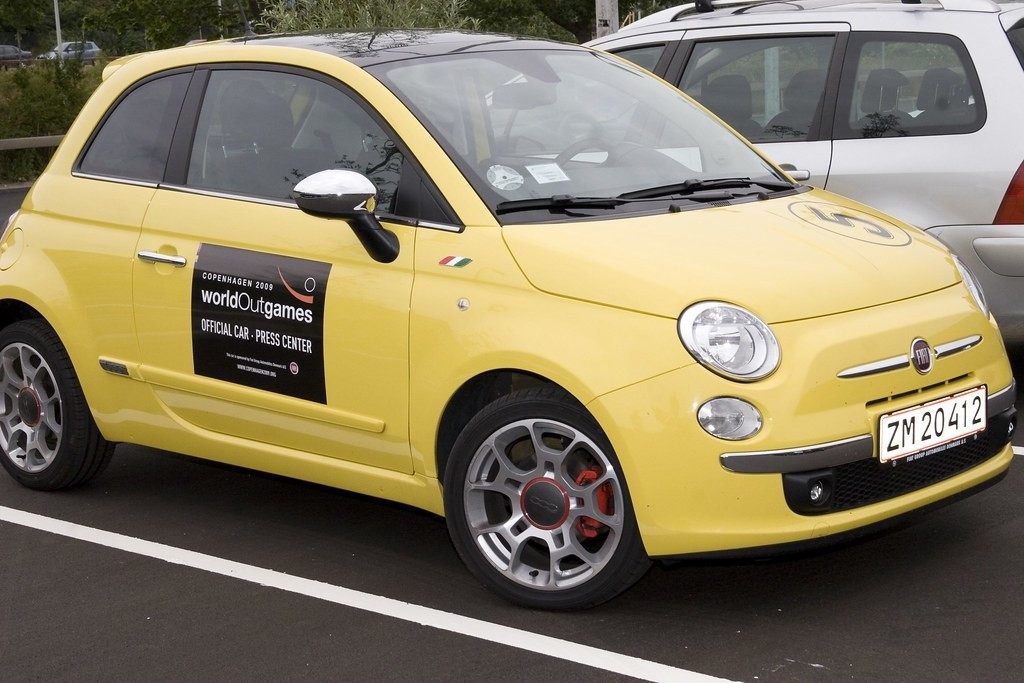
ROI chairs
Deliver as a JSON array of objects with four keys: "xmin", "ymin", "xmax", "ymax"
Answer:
[
  {"xmin": 700, "ymin": 69, "xmax": 827, "ymax": 141},
  {"xmin": 854, "ymin": 67, "xmax": 968, "ymax": 130},
  {"xmin": 215, "ymin": 94, "xmax": 322, "ymax": 198},
  {"xmin": 347, "ymin": 105, "xmax": 449, "ymax": 190}
]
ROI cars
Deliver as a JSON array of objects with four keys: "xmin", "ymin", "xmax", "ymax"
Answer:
[
  {"xmin": 0, "ymin": 29, "xmax": 1015, "ymax": 612},
  {"xmin": 36, "ymin": 41, "xmax": 103, "ymax": 60},
  {"xmin": 0, "ymin": 45, "xmax": 33, "ymax": 69}
]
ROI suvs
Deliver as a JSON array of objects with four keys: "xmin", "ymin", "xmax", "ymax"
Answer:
[{"xmin": 456, "ymin": 0, "xmax": 1024, "ymax": 367}]
